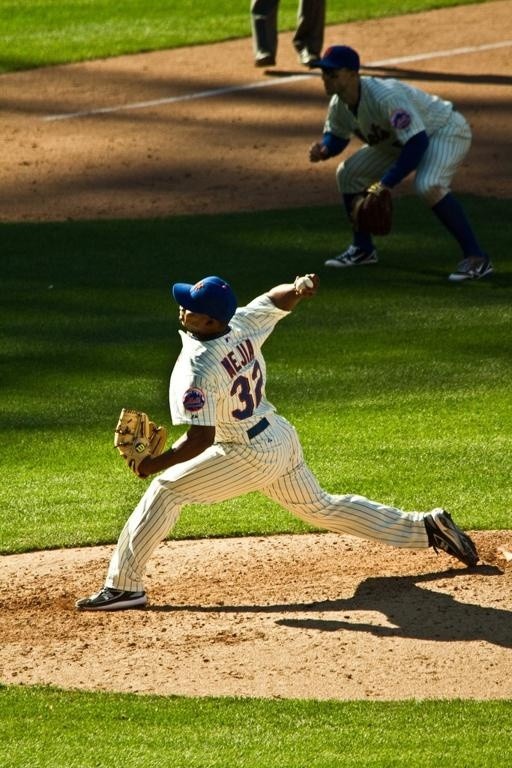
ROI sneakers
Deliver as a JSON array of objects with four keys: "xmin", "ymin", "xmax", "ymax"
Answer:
[
  {"xmin": 449, "ymin": 254, "xmax": 493, "ymax": 281},
  {"xmin": 423, "ymin": 507, "xmax": 479, "ymax": 567},
  {"xmin": 296, "ymin": 49, "xmax": 321, "ymax": 65},
  {"xmin": 254, "ymin": 54, "xmax": 276, "ymax": 68},
  {"xmin": 325, "ymin": 245, "xmax": 379, "ymax": 267},
  {"xmin": 74, "ymin": 586, "xmax": 148, "ymax": 612}
]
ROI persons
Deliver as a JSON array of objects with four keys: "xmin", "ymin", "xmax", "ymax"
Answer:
[
  {"xmin": 309, "ymin": 45, "xmax": 494, "ymax": 283},
  {"xmin": 75, "ymin": 272, "xmax": 479, "ymax": 611},
  {"xmin": 249, "ymin": 1, "xmax": 328, "ymax": 67}
]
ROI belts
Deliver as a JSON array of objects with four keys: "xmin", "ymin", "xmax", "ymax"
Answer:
[{"xmin": 248, "ymin": 416, "xmax": 270, "ymax": 440}]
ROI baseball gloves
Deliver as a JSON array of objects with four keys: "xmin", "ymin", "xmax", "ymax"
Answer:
[
  {"xmin": 352, "ymin": 179, "xmax": 391, "ymax": 235},
  {"xmin": 114, "ymin": 406, "xmax": 166, "ymax": 477}
]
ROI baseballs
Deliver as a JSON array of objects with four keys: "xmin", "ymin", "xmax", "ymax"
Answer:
[{"xmin": 295, "ymin": 276, "xmax": 314, "ymax": 292}]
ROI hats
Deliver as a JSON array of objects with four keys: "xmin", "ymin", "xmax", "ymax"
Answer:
[
  {"xmin": 172, "ymin": 275, "xmax": 238, "ymax": 324},
  {"xmin": 312, "ymin": 45, "xmax": 360, "ymax": 69}
]
